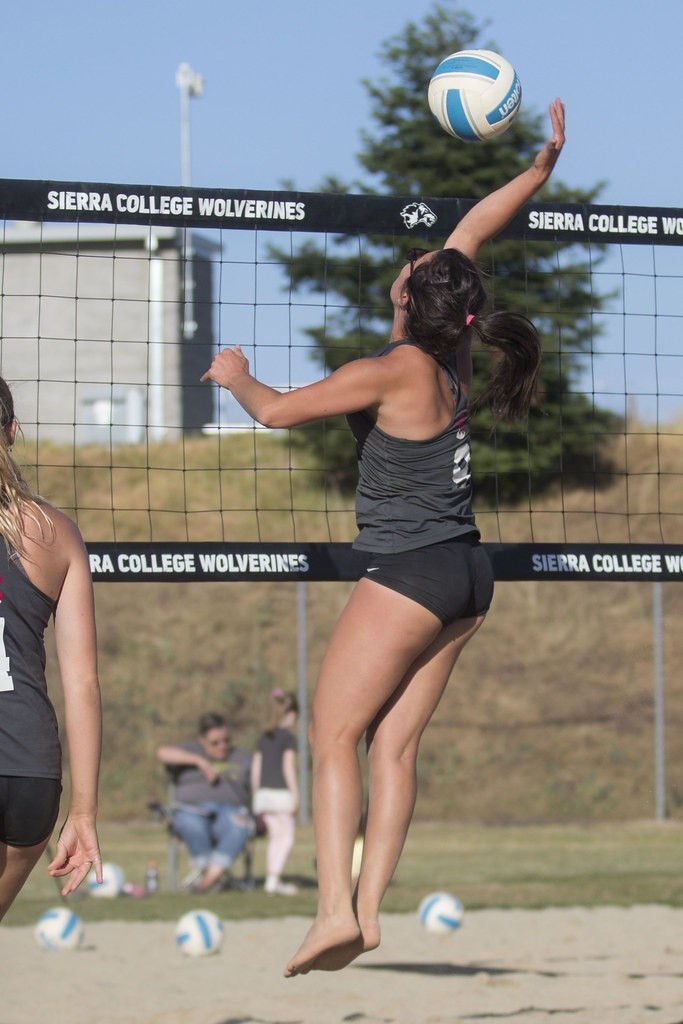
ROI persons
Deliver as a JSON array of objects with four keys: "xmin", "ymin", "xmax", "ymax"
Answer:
[
  {"xmin": 200, "ymin": 96, "xmax": 568, "ymax": 978},
  {"xmin": 1, "ymin": 377, "xmax": 107, "ymax": 924},
  {"xmin": 248, "ymin": 689, "xmax": 305, "ymax": 896},
  {"xmin": 156, "ymin": 709, "xmax": 256, "ymax": 893}
]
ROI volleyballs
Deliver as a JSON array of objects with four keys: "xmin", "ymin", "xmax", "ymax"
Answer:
[
  {"xmin": 35, "ymin": 908, "xmax": 84, "ymax": 951},
  {"xmin": 87, "ymin": 863, "xmax": 127, "ymax": 901},
  {"xmin": 428, "ymin": 48, "xmax": 524, "ymax": 144},
  {"xmin": 178, "ymin": 908, "xmax": 227, "ymax": 956},
  {"xmin": 416, "ymin": 892, "xmax": 463, "ymax": 935}
]
